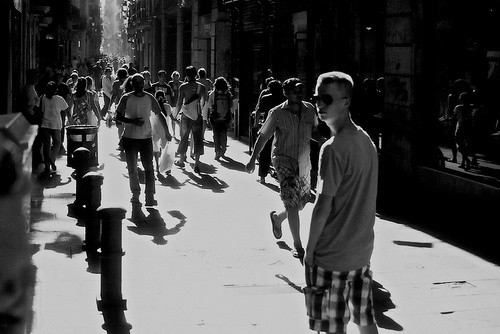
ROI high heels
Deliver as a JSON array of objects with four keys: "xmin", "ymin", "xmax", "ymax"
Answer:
[{"xmin": 215, "ymin": 149, "xmax": 225, "ymax": 161}]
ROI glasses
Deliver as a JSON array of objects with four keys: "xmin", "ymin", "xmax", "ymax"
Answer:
[{"xmin": 310, "ymin": 93, "xmax": 349, "ymax": 105}]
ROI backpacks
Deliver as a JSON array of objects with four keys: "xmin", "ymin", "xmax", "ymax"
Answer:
[{"xmin": 212, "ymin": 91, "xmax": 232, "ymax": 121}]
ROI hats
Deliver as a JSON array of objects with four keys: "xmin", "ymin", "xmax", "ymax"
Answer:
[{"xmin": 282, "ymin": 76, "xmax": 303, "ymax": 90}]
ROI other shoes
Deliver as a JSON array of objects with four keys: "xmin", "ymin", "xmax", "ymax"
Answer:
[
  {"xmin": 48, "ymin": 150, "xmax": 56, "ymax": 171},
  {"xmin": 194, "ymin": 166, "xmax": 200, "ymax": 173},
  {"xmin": 145, "ymin": 198, "xmax": 158, "ymax": 206},
  {"xmin": 130, "ymin": 196, "xmax": 142, "ymax": 207},
  {"xmin": 447, "ymin": 157, "xmax": 480, "ymax": 171}
]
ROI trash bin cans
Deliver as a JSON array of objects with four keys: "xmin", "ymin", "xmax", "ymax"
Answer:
[{"xmin": 66, "ymin": 127, "xmax": 98, "ymax": 168}]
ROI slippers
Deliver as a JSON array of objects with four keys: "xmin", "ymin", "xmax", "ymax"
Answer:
[
  {"xmin": 270, "ymin": 211, "xmax": 282, "ymax": 239},
  {"xmin": 291, "ymin": 243, "xmax": 305, "ymax": 258}
]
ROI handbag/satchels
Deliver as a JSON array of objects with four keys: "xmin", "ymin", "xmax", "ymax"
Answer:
[
  {"xmin": 159, "ymin": 138, "xmax": 176, "ymax": 173},
  {"xmin": 31, "ymin": 95, "xmax": 44, "ymax": 126},
  {"xmin": 174, "ymin": 159, "xmax": 185, "ymax": 167},
  {"xmin": 181, "ymin": 96, "xmax": 202, "ymax": 121}
]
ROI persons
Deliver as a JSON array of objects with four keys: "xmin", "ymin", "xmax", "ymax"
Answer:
[
  {"xmin": 303, "ymin": 71, "xmax": 379, "ymax": 334},
  {"xmin": 21, "ymin": 55, "xmax": 478, "ymax": 257}
]
